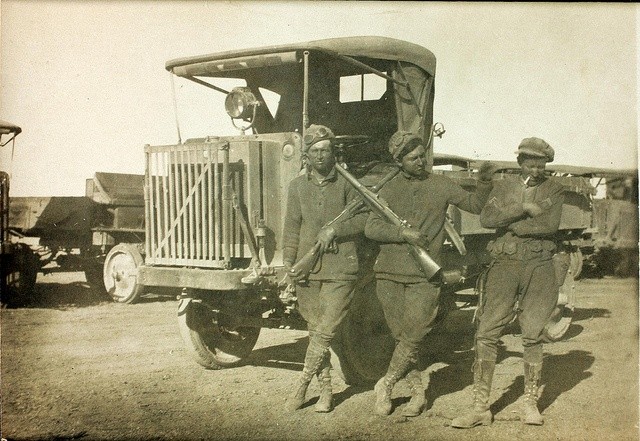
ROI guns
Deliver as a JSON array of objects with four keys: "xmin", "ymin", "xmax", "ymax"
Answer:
[
  {"xmin": 334, "ymin": 162, "xmax": 443, "ymax": 284},
  {"xmin": 289, "ymin": 167, "xmax": 400, "ymax": 287}
]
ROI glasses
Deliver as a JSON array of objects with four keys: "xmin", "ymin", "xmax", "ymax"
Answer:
[
  {"xmin": 302, "ymin": 128, "xmax": 328, "ymax": 144},
  {"xmin": 391, "ymin": 131, "xmax": 412, "ymax": 155}
]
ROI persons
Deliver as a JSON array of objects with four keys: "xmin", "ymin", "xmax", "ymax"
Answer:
[
  {"xmin": 449, "ymin": 137, "xmax": 568, "ymax": 428},
  {"xmin": 283, "ymin": 125, "xmax": 369, "ymax": 414},
  {"xmin": 364, "ymin": 130, "xmax": 499, "ymax": 419}
]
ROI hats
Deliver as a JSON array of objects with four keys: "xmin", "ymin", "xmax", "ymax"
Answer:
[
  {"xmin": 302, "ymin": 124, "xmax": 336, "ymax": 152},
  {"xmin": 514, "ymin": 137, "xmax": 554, "ymax": 162},
  {"xmin": 388, "ymin": 131, "xmax": 421, "ymax": 160}
]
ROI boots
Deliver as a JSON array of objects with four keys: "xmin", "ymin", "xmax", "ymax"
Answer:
[
  {"xmin": 309, "ymin": 336, "xmax": 335, "ymax": 413},
  {"xmin": 451, "ymin": 359, "xmax": 496, "ymax": 429},
  {"xmin": 400, "ymin": 353, "xmax": 428, "ymax": 417},
  {"xmin": 374, "ymin": 342, "xmax": 422, "ymax": 416},
  {"xmin": 519, "ymin": 359, "xmax": 544, "ymax": 426},
  {"xmin": 285, "ymin": 338, "xmax": 329, "ymax": 412}
]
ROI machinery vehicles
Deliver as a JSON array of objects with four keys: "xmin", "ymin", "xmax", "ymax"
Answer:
[
  {"xmin": 548, "ymin": 165, "xmax": 639, "ymax": 280},
  {"xmin": 138, "ymin": 36, "xmax": 592, "ymax": 385},
  {"xmin": 1, "ymin": 171, "xmax": 149, "ymax": 304}
]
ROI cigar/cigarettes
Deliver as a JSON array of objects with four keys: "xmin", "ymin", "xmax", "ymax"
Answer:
[{"xmin": 524, "ymin": 176, "xmax": 530, "ymax": 185}]
